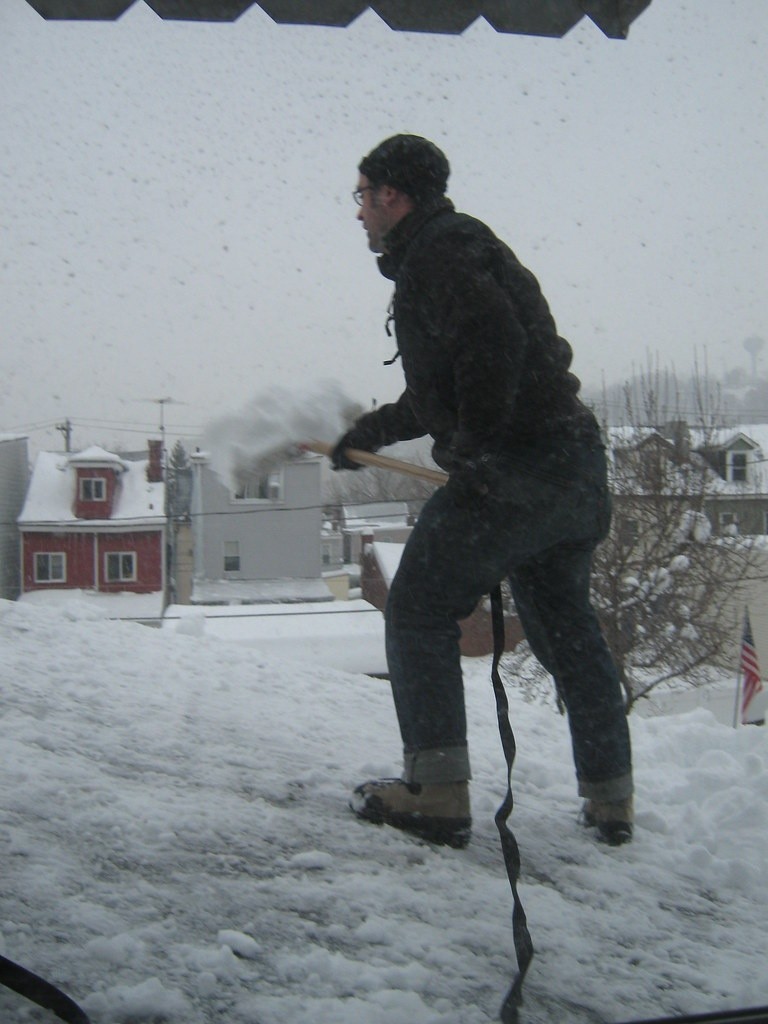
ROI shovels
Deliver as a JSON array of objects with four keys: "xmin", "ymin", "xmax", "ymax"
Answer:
[{"xmin": 221, "ymin": 398, "xmax": 449, "ymax": 487}]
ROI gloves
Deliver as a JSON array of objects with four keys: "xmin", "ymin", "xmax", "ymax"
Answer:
[
  {"xmin": 330, "ymin": 426, "xmax": 383, "ymax": 471},
  {"xmin": 446, "ymin": 465, "xmax": 495, "ymax": 507}
]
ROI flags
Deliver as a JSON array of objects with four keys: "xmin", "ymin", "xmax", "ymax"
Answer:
[{"xmin": 739, "ymin": 615, "xmax": 763, "ymax": 713}]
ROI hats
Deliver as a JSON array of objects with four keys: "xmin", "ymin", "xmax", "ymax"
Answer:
[{"xmin": 358, "ymin": 134, "xmax": 450, "ymax": 199}]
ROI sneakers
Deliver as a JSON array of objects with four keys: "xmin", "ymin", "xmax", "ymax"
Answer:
[
  {"xmin": 350, "ymin": 776, "xmax": 471, "ymax": 848},
  {"xmin": 583, "ymin": 797, "xmax": 635, "ymax": 846}
]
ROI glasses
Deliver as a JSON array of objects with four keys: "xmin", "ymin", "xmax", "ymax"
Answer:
[{"xmin": 353, "ymin": 184, "xmax": 380, "ymax": 206}]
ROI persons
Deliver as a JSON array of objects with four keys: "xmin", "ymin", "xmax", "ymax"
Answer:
[{"xmin": 329, "ymin": 134, "xmax": 632, "ymax": 847}]
